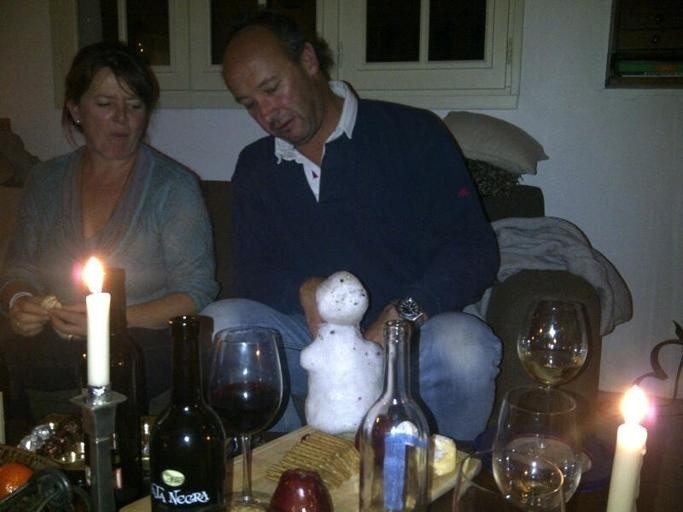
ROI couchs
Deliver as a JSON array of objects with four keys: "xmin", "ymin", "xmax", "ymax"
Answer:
[{"xmin": 0, "ymin": 180, "xmax": 601, "ymax": 512}]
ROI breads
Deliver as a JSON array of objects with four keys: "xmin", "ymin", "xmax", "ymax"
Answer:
[{"xmin": 428, "ymin": 433, "xmax": 457, "ymax": 477}]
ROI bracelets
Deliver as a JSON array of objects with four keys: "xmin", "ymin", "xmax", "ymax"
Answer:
[{"xmin": 3, "ymin": 288, "xmax": 37, "ymax": 316}]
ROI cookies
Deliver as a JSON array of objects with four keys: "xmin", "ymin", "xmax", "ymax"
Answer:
[{"xmin": 265, "ymin": 431, "xmax": 360, "ymax": 489}]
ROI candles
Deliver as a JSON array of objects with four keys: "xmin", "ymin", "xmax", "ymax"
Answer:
[
  {"xmin": 606, "ymin": 385, "xmax": 658, "ymax": 512},
  {"xmin": 81, "ymin": 255, "xmax": 112, "ymax": 388}
]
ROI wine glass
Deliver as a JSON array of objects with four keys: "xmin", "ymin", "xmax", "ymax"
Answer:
[{"xmin": 207, "ymin": 328, "xmax": 285, "ymax": 512}]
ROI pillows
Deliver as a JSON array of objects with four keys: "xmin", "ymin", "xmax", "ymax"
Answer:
[{"xmin": 444, "ymin": 112, "xmax": 550, "ymax": 176}]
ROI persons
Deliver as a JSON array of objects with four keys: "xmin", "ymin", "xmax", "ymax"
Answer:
[
  {"xmin": 0, "ymin": 30, "xmax": 217, "ymax": 434},
  {"xmin": 196, "ymin": 13, "xmax": 501, "ymax": 449}
]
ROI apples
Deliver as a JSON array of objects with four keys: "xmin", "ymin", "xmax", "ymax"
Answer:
[{"xmin": 270, "ymin": 469, "xmax": 333, "ymax": 512}]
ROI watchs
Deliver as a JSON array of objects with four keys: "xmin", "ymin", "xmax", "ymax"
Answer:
[{"xmin": 392, "ymin": 293, "xmax": 424, "ymax": 344}]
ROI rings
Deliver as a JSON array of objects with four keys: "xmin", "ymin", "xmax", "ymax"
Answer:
[
  {"xmin": 13, "ymin": 320, "xmax": 20, "ymax": 330},
  {"xmin": 66, "ymin": 333, "xmax": 73, "ymax": 344}
]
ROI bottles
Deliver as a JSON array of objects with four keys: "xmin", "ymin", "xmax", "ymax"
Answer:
[
  {"xmin": 148, "ymin": 315, "xmax": 224, "ymax": 511},
  {"xmin": 360, "ymin": 321, "xmax": 429, "ymax": 509}
]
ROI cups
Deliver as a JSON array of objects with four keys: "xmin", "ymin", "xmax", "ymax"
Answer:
[{"xmin": 453, "ymin": 299, "xmax": 587, "ymax": 511}]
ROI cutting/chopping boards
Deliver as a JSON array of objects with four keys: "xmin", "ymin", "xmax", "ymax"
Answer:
[{"xmin": 124, "ymin": 425, "xmax": 479, "ymax": 511}]
